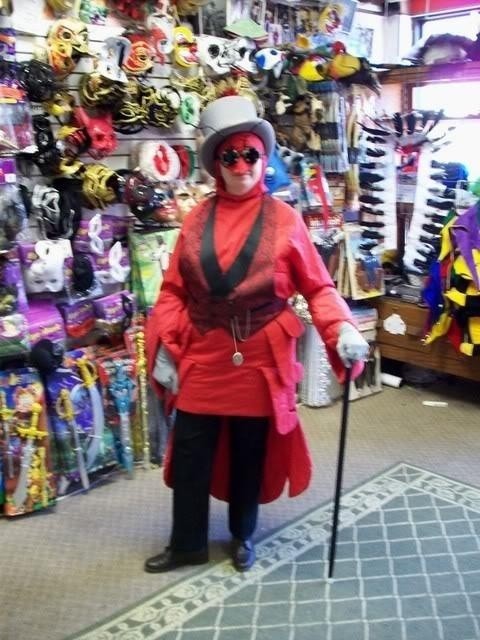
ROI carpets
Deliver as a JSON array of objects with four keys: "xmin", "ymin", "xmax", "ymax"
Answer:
[{"xmin": 56, "ymin": 462, "xmax": 478, "ymax": 640}]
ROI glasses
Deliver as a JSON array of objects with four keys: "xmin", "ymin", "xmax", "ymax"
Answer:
[{"xmin": 214, "ymin": 148, "xmax": 263, "ymax": 164}]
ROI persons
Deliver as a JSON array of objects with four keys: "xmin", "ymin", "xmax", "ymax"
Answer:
[{"xmin": 144, "ymin": 95, "xmax": 372, "ymax": 573}]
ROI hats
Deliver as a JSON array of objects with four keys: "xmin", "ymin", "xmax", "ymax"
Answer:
[{"xmin": 199, "ymin": 95, "xmax": 276, "ymax": 178}]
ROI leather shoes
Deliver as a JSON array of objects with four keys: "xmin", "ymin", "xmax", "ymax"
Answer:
[
  {"xmin": 145, "ymin": 546, "xmax": 209, "ymax": 573},
  {"xmin": 231, "ymin": 536, "xmax": 256, "ymax": 572}
]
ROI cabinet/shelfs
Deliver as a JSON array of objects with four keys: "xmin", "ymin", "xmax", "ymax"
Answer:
[{"xmin": 377, "ymin": 301, "xmax": 479, "ymax": 386}]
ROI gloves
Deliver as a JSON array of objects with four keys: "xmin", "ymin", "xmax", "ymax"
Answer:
[
  {"xmin": 152, "ymin": 344, "xmax": 178, "ymax": 395},
  {"xmin": 336, "ymin": 320, "xmax": 370, "ymax": 368}
]
